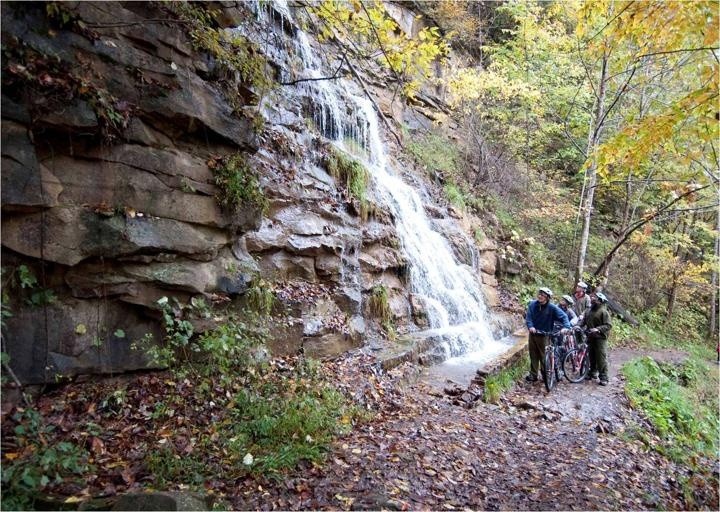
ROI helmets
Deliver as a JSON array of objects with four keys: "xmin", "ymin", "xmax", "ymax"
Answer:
[
  {"xmin": 562, "ymin": 295, "xmax": 573, "ymax": 304},
  {"xmin": 577, "ymin": 282, "xmax": 587, "ymax": 289},
  {"xmin": 594, "ymin": 293, "xmax": 608, "ymax": 303},
  {"xmin": 539, "ymin": 286, "xmax": 552, "ymax": 299}
]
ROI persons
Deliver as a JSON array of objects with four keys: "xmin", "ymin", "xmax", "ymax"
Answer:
[{"xmin": 526, "ymin": 282, "xmax": 612, "ymax": 386}]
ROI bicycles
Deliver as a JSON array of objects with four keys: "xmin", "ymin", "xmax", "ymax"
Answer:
[{"xmin": 534, "ymin": 326, "xmax": 602, "ymax": 393}]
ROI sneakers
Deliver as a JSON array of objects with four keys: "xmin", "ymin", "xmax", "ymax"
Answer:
[
  {"xmin": 600, "ymin": 379, "xmax": 608, "ymax": 386},
  {"xmin": 526, "ymin": 374, "xmax": 538, "ymax": 381},
  {"xmin": 584, "ymin": 372, "xmax": 597, "ymax": 380}
]
